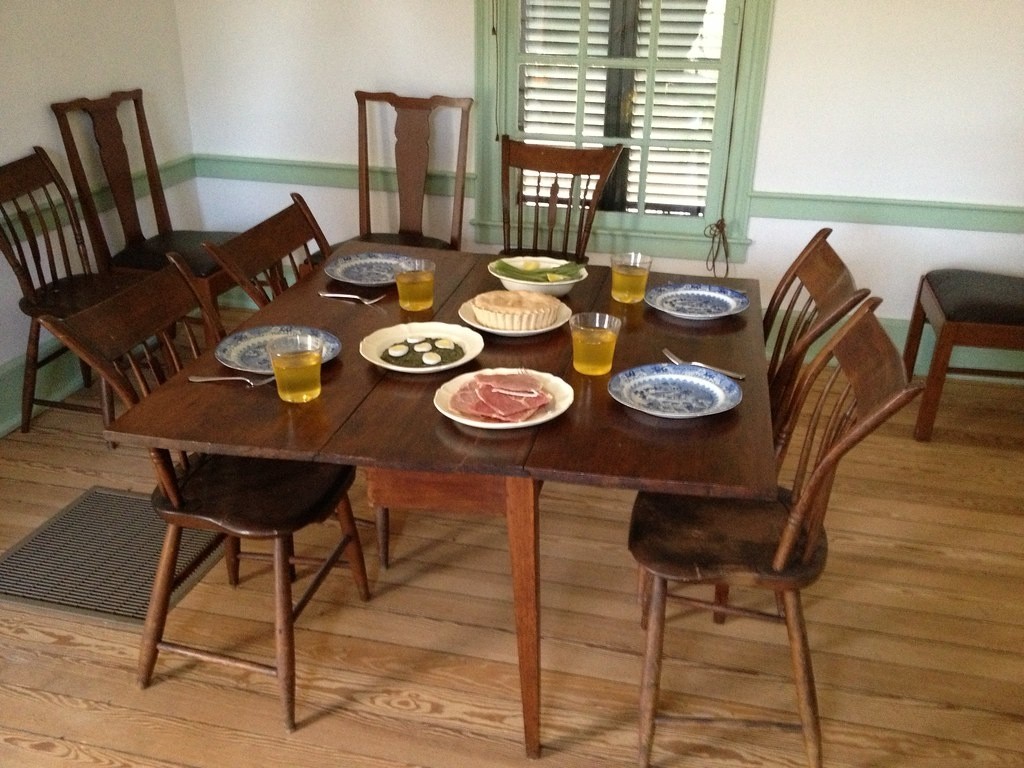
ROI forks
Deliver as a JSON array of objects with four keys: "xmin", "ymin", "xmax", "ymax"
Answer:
[
  {"xmin": 187, "ymin": 375, "xmax": 275, "ymax": 386},
  {"xmin": 662, "ymin": 348, "xmax": 746, "ymax": 379},
  {"xmin": 319, "ymin": 291, "xmax": 387, "ymax": 304}
]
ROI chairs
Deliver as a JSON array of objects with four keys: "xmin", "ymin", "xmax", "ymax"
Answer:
[
  {"xmin": 199, "ymin": 192, "xmax": 388, "ymax": 572},
  {"xmin": 36, "ymin": 253, "xmax": 373, "ymax": 735},
  {"xmin": 497, "ymin": 133, "xmax": 628, "ymax": 263},
  {"xmin": 631, "ymin": 298, "xmax": 917, "ymax": 768},
  {"xmin": 50, "ymin": 89, "xmax": 285, "ymax": 352},
  {"xmin": 298, "ymin": 90, "xmax": 474, "ymax": 275},
  {"xmin": 0, "ymin": 146, "xmax": 172, "ymax": 451},
  {"xmin": 639, "ymin": 227, "xmax": 869, "ymax": 626}
]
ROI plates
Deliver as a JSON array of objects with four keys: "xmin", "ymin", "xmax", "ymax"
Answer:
[
  {"xmin": 214, "ymin": 324, "xmax": 343, "ymax": 374},
  {"xmin": 641, "ymin": 305, "xmax": 748, "ymax": 340},
  {"xmin": 644, "ymin": 282, "xmax": 750, "ymax": 321},
  {"xmin": 457, "ymin": 299, "xmax": 572, "ymax": 337},
  {"xmin": 324, "ymin": 252, "xmax": 404, "ymax": 286},
  {"xmin": 359, "ymin": 321, "xmax": 484, "ymax": 373},
  {"xmin": 607, "ymin": 364, "xmax": 743, "ymax": 419},
  {"xmin": 432, "ymin": 366, "xmax": 576, "ymax": 429}
]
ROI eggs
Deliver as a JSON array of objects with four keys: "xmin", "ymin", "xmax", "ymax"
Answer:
[{"xmin": 388, "ymin": 336, "xmax": 455, "ymax": 365}]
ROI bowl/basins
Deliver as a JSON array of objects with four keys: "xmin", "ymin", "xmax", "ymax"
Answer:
[{"xmin": 487, "ymin": 256, "xmax": 589, "ymax": 297}]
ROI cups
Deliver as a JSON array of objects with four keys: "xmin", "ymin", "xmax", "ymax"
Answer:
[
  {"xmin": 610, "ymin": 252, "xmax": 652, "ymax": 303},
  {"xmin": 266, "ymin": 335, "xmax": 323, "ymax": 403},
  {"xmin": 392, "ymin": 258, "xmax": 436, "ymax": 311},
  {"xmin": 569, "ymin": 313, "xmax": 621, "ymax": 375}
]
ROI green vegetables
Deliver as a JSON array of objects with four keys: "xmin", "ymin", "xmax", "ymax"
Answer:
[{"xmin": 491, "ymin": 259, "xmax": 587, "ymax": 282}]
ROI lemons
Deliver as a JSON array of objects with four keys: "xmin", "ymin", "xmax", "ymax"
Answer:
[
  {"xmin": 524, "ymin": 261, "xmax": 539, "ymax": 270},
  {"xmin": 546, "ymin": 273, "xmax": 572, "ymax": 282}
]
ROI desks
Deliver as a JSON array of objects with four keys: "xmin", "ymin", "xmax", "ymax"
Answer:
[{"xmin": 105, "ymin": 239, "xmax": 777, "ymax": 760}]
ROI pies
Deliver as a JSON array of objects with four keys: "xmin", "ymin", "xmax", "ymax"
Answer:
[{"xmin": 471, "ymin": 290, "xmax": 561, "ymax": 330}]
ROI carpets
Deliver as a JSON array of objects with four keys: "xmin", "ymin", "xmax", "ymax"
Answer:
[{"xmin": 0, "ymin": 485, "xmax": 222, "ymax": 625}]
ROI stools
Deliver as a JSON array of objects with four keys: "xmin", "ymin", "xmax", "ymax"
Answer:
[{"xmin": 900, "ymin": 270, "xmax": 1024, "ymax": 441}]
